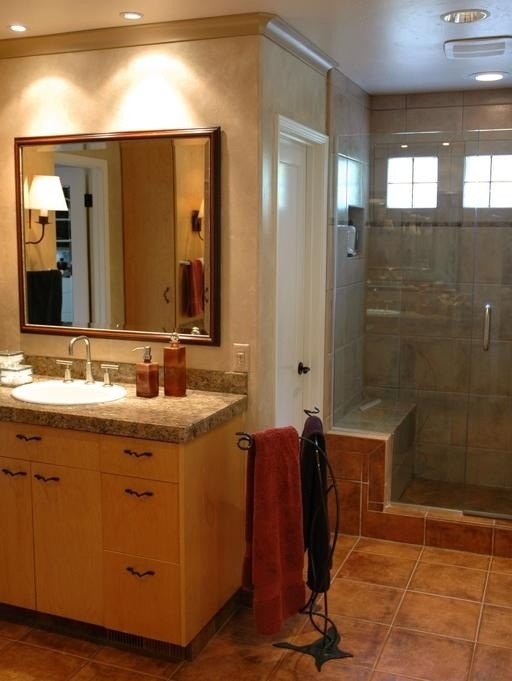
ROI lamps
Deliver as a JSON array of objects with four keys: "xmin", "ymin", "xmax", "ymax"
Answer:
[{"xmin": 25, "ymin": 175, "xmax": 69, "ymax": 245}]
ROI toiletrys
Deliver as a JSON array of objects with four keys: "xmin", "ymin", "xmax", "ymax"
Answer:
[
  {"xmin": 162, "ymin": 333, "xmax": 186, "ymax": 398},
  {"xmin": 130, "ymin": 345, "xmax": 159, "ymax": 399},
  {"xmin": 190, "ymin": 327, "xmax": 200, "ymax": 336}
]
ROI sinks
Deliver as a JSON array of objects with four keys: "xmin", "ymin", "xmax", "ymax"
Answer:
[{"xmin": 11, "ymin": 379, "xmax": 127, "ymax": 405}]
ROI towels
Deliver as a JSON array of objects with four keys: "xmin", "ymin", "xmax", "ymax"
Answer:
[
  {"xmin": 300, "ymin": 415, "xmax": 332, "ymax": 594},
  {"xmin": 182, "ymin": 258, "xmax": 203, "ymax": 318},
  {"xmin": 242, "ymin": 425, "xmax": 307, "ymax": 638}
]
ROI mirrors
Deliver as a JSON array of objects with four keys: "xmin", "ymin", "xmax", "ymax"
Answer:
[{"xmin": 13, "ymin": 127, "xmax": 223, "ymax": 346}]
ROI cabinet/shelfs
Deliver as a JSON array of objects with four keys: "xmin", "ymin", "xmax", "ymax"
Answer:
[
  {"xmin": 0, "ymin": 421, "xmax": 98, "ymax": 628},
  {"xmin": 98, "ymin": 413, "xmax": 245, "ymax": 664}
]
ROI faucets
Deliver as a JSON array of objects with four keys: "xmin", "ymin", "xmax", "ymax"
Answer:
[{"xmin": 68, "ymin": 334, "xmax": 95, "ymax": 384}]
